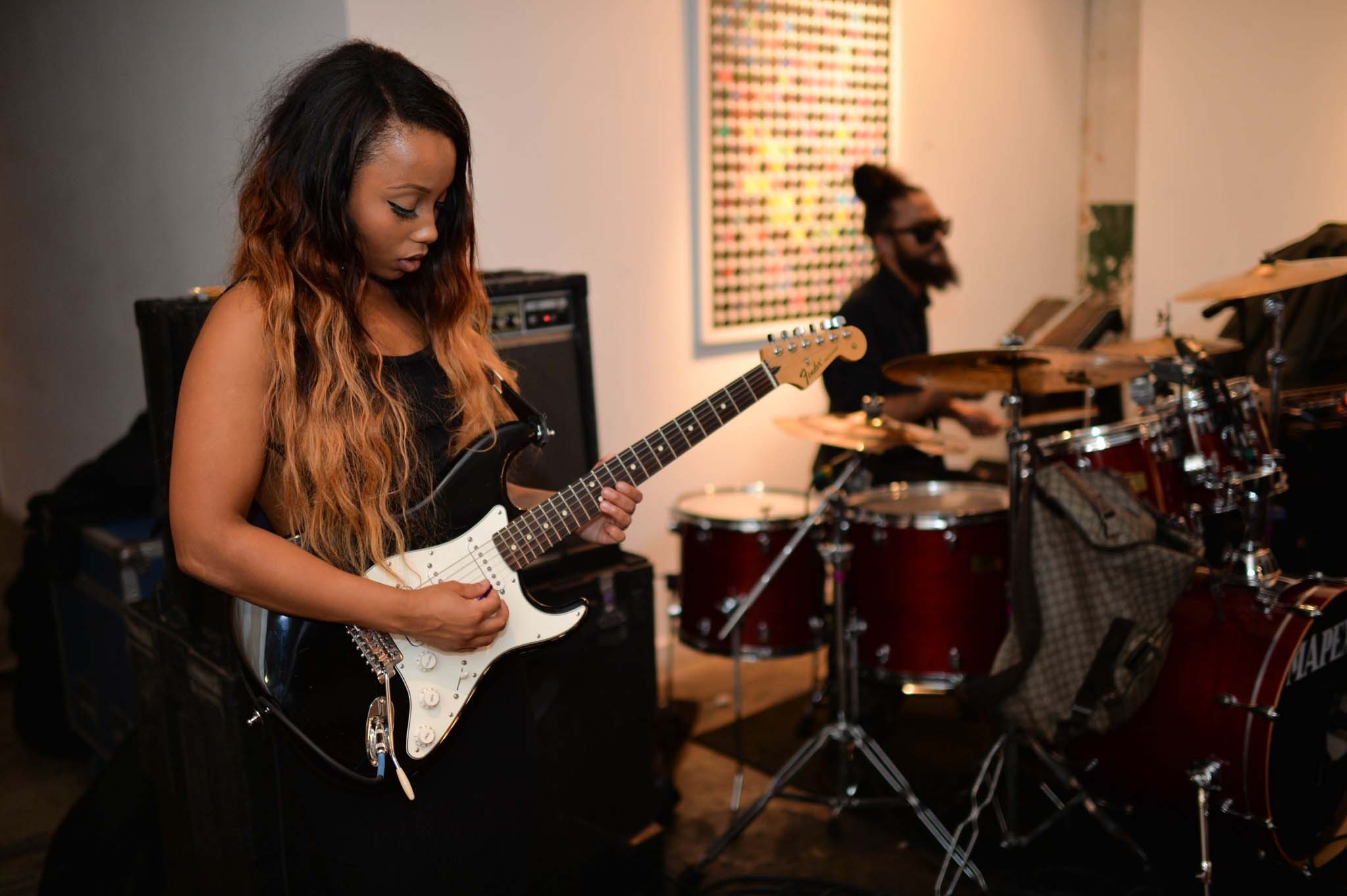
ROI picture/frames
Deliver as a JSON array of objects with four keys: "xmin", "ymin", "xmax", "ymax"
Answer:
[{"xmin": 692, "ymin": 1, "xmax": 911, "ymax": 360}]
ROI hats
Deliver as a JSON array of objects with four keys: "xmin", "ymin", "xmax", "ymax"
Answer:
[{"xmin": 853, "ymin": 165, "xmax": 912, "ymax": 227}]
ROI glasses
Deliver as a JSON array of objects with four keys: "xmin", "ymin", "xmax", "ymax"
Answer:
[{"xmin": 866, "ymin": 218, "xmax": 952, "ymax": 247}]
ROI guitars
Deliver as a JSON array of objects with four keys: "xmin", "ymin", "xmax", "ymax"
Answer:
[{"xmin": 284, "ymin": 312, "xmax": 869, "ymax": 771}]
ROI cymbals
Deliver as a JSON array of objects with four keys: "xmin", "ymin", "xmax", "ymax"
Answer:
[
  {"xmin": 880, "ymin": 338, "xmax": 1153, "ymax": 398},
  {"xmin": 768, "ymin": 399, "xmax": 969, "ymax": 459},
  {"xmin": 1095, "ymin": 331, "xmax": 1245, "ymax": 366},
  {"xmin": 1172, "ymin": 254, "xmax": 1347, "ymax": 302}
]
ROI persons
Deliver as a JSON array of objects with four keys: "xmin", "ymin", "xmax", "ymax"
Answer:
[
  {"xmin": 813, "ymin": 164, "xmax": 980, "ymax": 488},
  {"xmin": 164, "ymin": 43, "xmax": 650, "ymax": 895}
]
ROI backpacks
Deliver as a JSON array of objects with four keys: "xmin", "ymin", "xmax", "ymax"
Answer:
[{"xmin": 985, "ymin": 442, "xmax": 1206, "ymax": 751}]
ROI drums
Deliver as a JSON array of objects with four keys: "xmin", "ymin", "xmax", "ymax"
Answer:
[
  {"xmin": 1038, "ymin": 374, "xmax": 1295, "ymax": 570},
  {"xmin": 1152, "ymin": 535, "xmax": 1345, "ymax": 879},
  {"xmin": 664, "ymin": 482, "xmax": 833, "ymax": 665},
  {"xmin": 838, "ymin": 475, "xmax": 1015, "ymax": 689}
]
